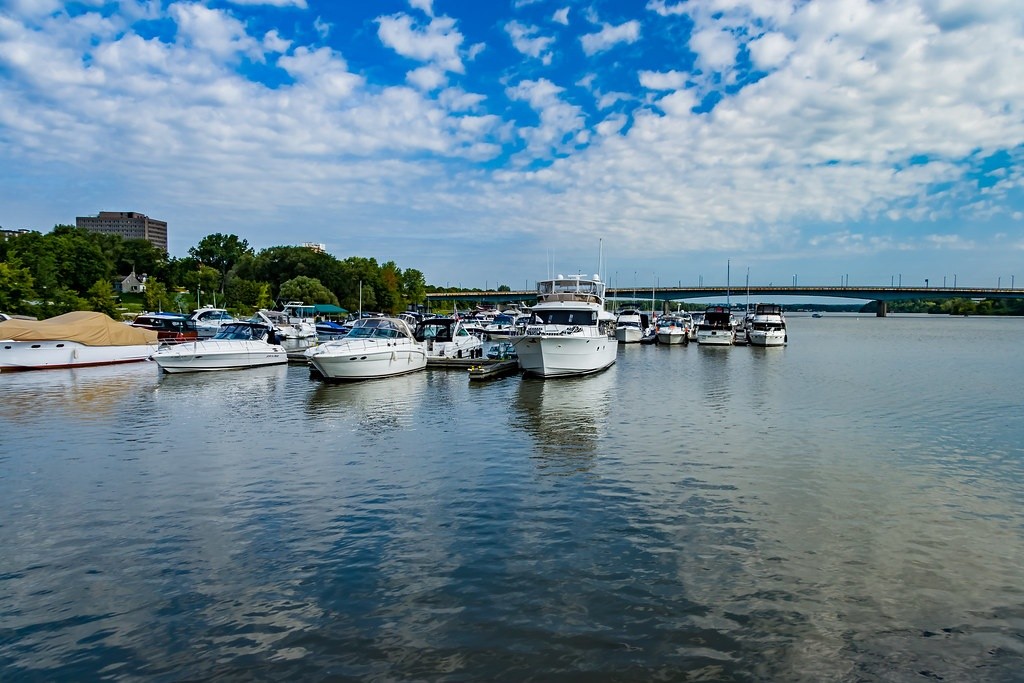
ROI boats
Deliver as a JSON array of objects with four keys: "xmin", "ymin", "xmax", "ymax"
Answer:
[
  {"xmin": 187, "ymin": 305, "xmax": 238, "ymax": 337},
  {"xmin": 128, "ymin": 313, "xmax": 199, "ymax": 345},
  {"xmin": 0, "ymin": 309, "xmax": 157, "ymax": 369},
  {"xmin": 152, "ymin": 321, "xmax": 288, "ymax": 374},
  {"xmin": 393, "ymin": 233, "xmax": 789, "ymax": 382},
  {"xmin": 246, "ymin": 302, "xmax": 316, "ymax": 340},
  {"xmin": 313, "ymin": 320, "xmax": 346, "ymax": 336},
  {"xmin": 304, "ymin": 280, "xmax": 429, "ymax": 380}
]
